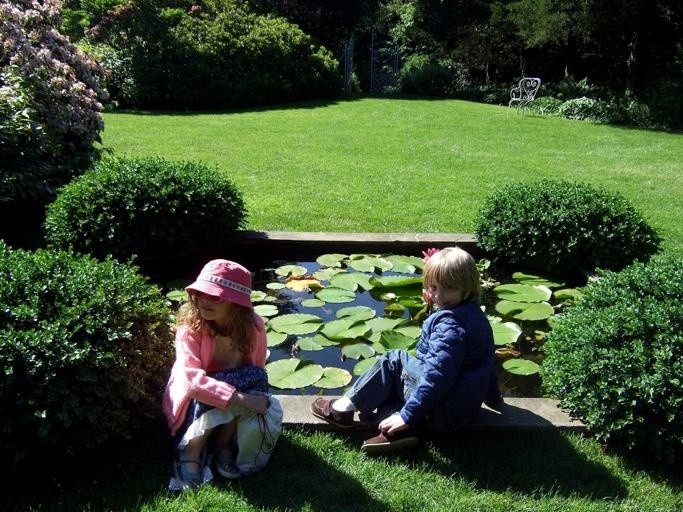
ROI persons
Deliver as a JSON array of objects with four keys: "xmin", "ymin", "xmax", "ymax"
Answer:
[
  {"xmin": 307, "ymin": 245, "xmax": 504, "ymax": 455},
  {"xmin": 161, "ymin": 257, "xmax": 284, "ymax": 496}
]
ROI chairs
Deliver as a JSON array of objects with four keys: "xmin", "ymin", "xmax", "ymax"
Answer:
[{"xmin": 506, "ymin": 77, "xmax": 543, "ymax": 118}]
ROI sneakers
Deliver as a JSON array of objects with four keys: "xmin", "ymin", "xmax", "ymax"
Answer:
[
  {"xmin": 310, "ymin": 397, "xmax": 356, "ymax": 431},
  {"xmin": 207, "ymin": 440, "xmax": 241, "ymax": 481},
  {"xmin": 170, "ymin": 453, "xmax": 202, "ymax": 495},
  {"xmin": 359, "ymin": 427, "xmax": 420, "ymax": 457}
]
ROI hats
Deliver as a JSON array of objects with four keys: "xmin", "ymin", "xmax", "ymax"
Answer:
[{"xmin": 182, "ymin": 258, "xmax": 254, "ymax": 311}]
